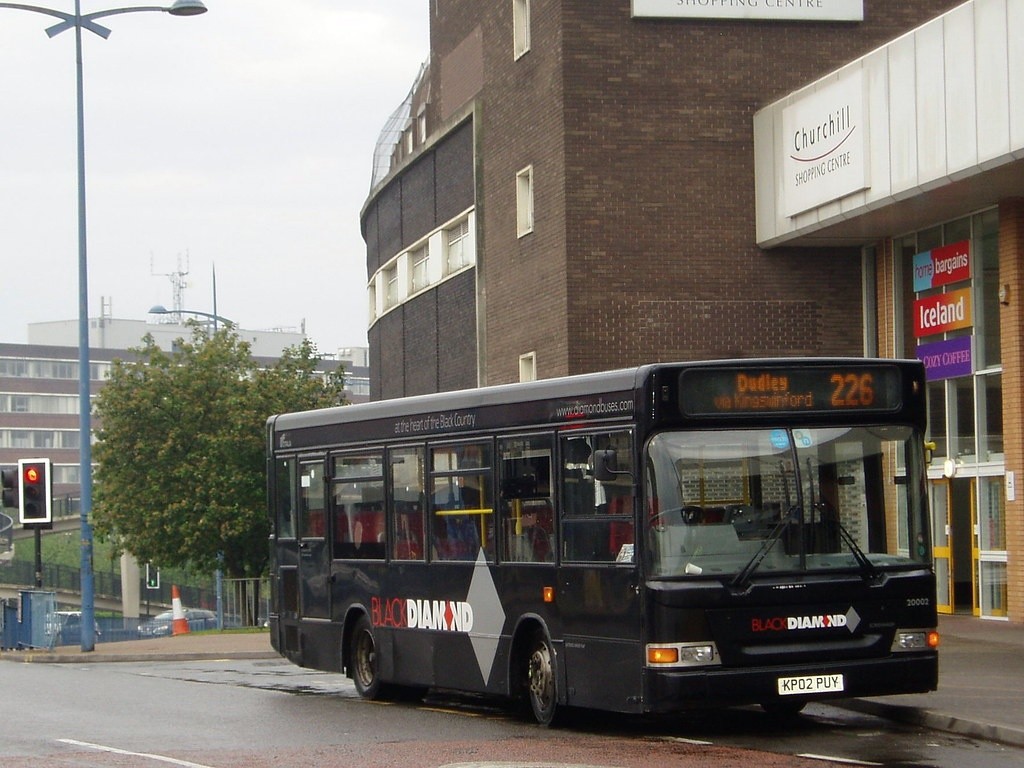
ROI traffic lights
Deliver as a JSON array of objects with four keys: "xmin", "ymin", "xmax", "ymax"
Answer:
[
  {"xmin": 1, "ymin": 466, "xmax": 20, "ymax": 508},
  {"xmin": 18, "ymin": 457, "xmax": 52, "ymax": 524},
  {"xmin": 145, "ymin": 558, "xmax": 160, "ymax": 591}
]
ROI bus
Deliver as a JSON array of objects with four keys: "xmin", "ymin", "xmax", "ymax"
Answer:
[{"xmin": 267, "ymin": 355, "xmax": 942, "ymax": 732}]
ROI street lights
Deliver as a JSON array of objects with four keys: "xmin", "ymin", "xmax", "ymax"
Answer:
[
  {"xmin": 0, "ymin": 0, "xmax": 210, "ymax": 653},
  {"xmin": 150, "ymin": 263, "xmax": 233, "ymax": 337}
]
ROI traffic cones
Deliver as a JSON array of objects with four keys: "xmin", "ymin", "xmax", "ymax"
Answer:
[{"xmin": 169, "ymin": 584, "xmax": 191, "ymax": 637}]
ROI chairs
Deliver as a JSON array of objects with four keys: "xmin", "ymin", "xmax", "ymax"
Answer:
[{"xmin": 310, "ymin": 496, "xmax": 727, "ymax": 561}]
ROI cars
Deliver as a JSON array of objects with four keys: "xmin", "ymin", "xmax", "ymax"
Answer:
[
  {"xmin": 46, "ymin": 611, "xmax": 102, "ymax": 649},
  {"xmin": 134, "ymin": 609, "xmax": 217, "ymax": 643}
]
